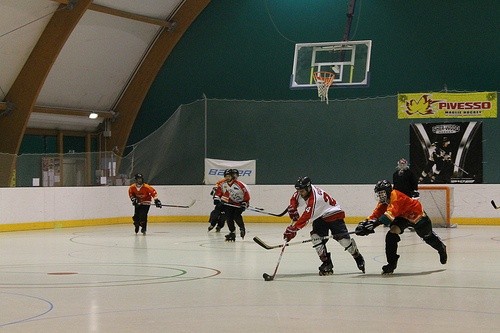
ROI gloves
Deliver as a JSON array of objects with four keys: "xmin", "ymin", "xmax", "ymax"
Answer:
[
  {"xmin": 210, "ymin": 187, "xmax": 217, "ymax": 196},
  {"xmin": 282, "ymin": 226, "xmax": 300, "ymax": 243},
  {"xmin": 355, "ymin": 219, "xmax": 379, "ymax": 236},
  {"xmin": 239, "ymin": 201, "xmax": 249, "ymax": 211},
  {"xmin": 213, "ymin": 195, "xmax": 221, "ymax": 205},
  {"xmin": 154, "ymin": 198, "xmax": 162, "ymax": 209},
  {"xmin": 131, "ymin": 198, "xmax": 139, "ymax": 207},
  {"xmin": 287, "ymin": 205, "xmax": 299, "ymax": 222}
]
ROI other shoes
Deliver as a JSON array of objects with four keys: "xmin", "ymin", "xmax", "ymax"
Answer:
[
  {"xmin": 430, "ymin": 175, "xmax": 436, "ymax": 183},
  {"xmin": 418, "ymin": 175, "xmax": 424, "ymax": 183},
  {"xmin": 135, "ymin": 228, "xmax": 139, "ymax": 234},
  {"xmin": 207, "ymin": 226, "xmax": 214, "ymax": 232},
  {"xmin": 216, "ymin": 226, "xmax": 221, "ymax": 232},
  {"xmin": 140, "ymin": 228, "xmax": 147, "ymax": 236}
]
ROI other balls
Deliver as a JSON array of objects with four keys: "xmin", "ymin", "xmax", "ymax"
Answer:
[{"xmin": 265, "ymin": 275, "xmax": 271, "ymax": 281}]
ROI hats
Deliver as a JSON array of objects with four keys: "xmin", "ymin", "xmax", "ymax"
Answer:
[{"xmin": 441, "ymin": 136, "xmax": 449, "ymax": 144}]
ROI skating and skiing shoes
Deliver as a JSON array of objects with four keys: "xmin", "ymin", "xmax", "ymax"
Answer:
[
  {"xmin": 438, "ymin": 247, "xmax": 449, "ymax": 265},
  {"xmin": 354, "ymin": 254, "xmax": 366, "ymax": 274},
  {"xmin": 316, "ymin": 252, "xmax": 334, "ymax": 276},
  {"xmin": 239, "ymin": 227, "xmax": 245, "ymax": 240},
  {"xmin": 223, "ymin": 232, "xmax": 236, "ymax": 242},
  {"xmin": 381, "ymin": 264, "xmax": 395, "ymax": 278}
]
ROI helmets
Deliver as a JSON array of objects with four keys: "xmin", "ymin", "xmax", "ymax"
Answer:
[
  {"xmin": 224, "ymin": 167, "xmax": 239, "ymax": 177},
  {"xmin": 135, "ymin": 173, "xmax": 143, "ymax": 179},
  {"xmin": 373, "ymin": 179, "xmax": 393, "ymax": 198},
  {"xmin": 294, "ymin": 176, "xmax": 312, "ymax": 189}
]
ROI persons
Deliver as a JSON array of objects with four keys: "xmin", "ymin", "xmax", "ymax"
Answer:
[
  {"xmin": 419, "ymin": 136, "xmax": 456, "ymax": 186},
  {"xmin": 129, "ymin": 174, "xmax": 162, "ymax": 234},
  {"xmin": 354, "ymin": 179, "xmax": 447, "ymax": 276},
  {"xmin": 393, "ymin": 158, "xmax": 421, "ymax": 232},
  {"xmin": 207, "ymin": 169, "xmax": 250, "ymax": 243},
  {"xmin": 284, "ymin": 175, "xmax": 365, "ymax": 276}
]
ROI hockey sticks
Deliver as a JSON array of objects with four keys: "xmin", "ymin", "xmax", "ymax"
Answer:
[
  {"xmin": 221, "ymin": 202, "xmax": 288, "ymax": 217},
  {"xmin": 137, "ymin": 200, "xmax": 196, "ymax": 208},
  {"xmin": 249, "ymin": 205, "xmax": 264, "ymax": 210},
  {"xmin": 253, "ymin": 230, "xmax": 364, "ymax": 250},
  {"xmin": 263, "ymin": 218, "xmax": 293, "ymax": 280},
  {"xmin": 491, "ymin": 200, "xmax": 500, "ymax": 209}
]
